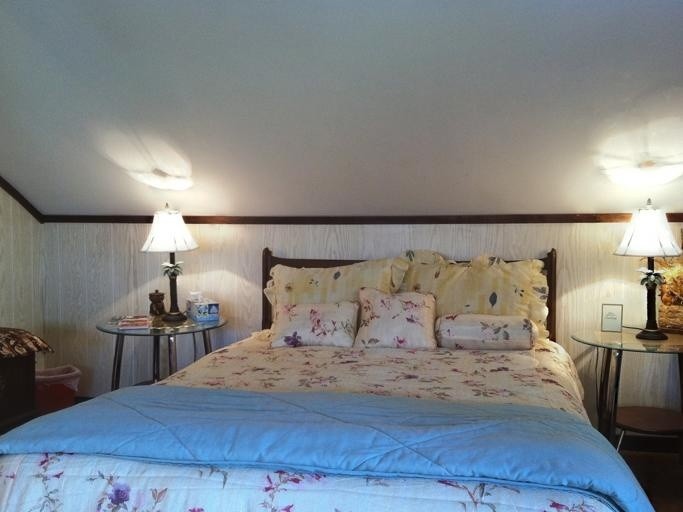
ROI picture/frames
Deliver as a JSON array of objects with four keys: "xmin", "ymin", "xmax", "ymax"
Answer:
[{"xmin": 601, "ymin": 303, "xmax": 623, "ymax": 332}]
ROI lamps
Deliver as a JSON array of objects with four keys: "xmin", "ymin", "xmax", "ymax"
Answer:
[
  {"xmin": 613, "ymin": 198, "xmax": 682, "ymax": 339},
  {"xmin": 140, "ymin": 202, "xmax": 198, "ymax": 322}
]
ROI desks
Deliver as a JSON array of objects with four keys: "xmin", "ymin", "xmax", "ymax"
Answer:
[
  {"xmin": 94, "ymin": 313, "xmax": 228, "ymax": 392},
  {"xmin": 569, "ymin": 328, "xmax": 683, "ymax": 454}
]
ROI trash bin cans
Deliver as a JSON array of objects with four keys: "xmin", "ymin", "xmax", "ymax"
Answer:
[{"xmin": 35, "ymin": 364, "xmax": 81, "ymax": 418}]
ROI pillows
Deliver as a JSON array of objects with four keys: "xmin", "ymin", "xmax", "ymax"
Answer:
[{"xmin": 263, "ymin": 249, "xmax": 550, "ymax": 351}]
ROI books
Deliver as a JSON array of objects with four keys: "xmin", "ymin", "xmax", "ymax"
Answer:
[{"xmin": 107, "ymin": 314, "xmax": 152, "ymax": 330}]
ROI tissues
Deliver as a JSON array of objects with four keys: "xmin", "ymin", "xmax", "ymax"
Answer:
[{"xmin": 185, "ymin": 289, "xmax": 219, "ymax": 322}]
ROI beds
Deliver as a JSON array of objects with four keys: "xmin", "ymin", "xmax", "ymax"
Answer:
[{"xmin": 0, "ymin": 247, "xmax": 624, "ymax": 512}]
